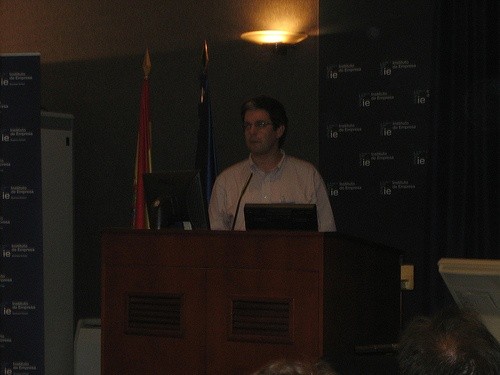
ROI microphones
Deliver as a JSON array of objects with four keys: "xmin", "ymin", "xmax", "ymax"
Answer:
[{"xmin": 231, "ymin": 172, "xmax": 253, "ymax": 230}]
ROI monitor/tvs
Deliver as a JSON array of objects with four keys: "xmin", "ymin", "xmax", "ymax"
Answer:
[{"xmin": 140, "ymin": 171, "xmax": 209, "ymax": 230}]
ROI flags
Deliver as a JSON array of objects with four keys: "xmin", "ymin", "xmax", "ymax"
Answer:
[
  {"xmin": 195, "ymin": 81, "xmax": 216, "ymax": 209},
  {"xmin": 129, "ymin": 80, "xmax": 151, "ymax": 228}
]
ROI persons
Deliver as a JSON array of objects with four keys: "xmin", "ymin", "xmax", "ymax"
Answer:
[
  {"xmin": 398, "ymin": 306, "xmax": 500, "ymax": 375},
  {"xmin": 209, "ymin": 96, "xmax": 337, "ymax": 232}
]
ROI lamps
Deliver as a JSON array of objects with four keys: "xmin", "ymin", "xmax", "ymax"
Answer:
[{"xmin": 240, "ymin": 29, "xmax": 308, "ymax": 51}]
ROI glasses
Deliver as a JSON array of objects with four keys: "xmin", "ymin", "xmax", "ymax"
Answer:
[{"xmin": 243, "ymin": 120, "xmax": 275, "ymax": 128}]
